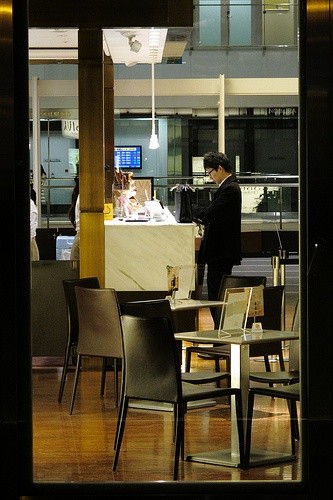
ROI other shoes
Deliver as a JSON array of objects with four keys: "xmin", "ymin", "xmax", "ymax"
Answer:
[{"xmin": 197, "ymin": 352, "xmax": 226, "ymax": 360}]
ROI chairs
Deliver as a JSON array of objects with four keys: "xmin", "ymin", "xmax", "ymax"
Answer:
[{"xmin": 58, "ymin": 274, "xmax": 301, "ymax": 481}]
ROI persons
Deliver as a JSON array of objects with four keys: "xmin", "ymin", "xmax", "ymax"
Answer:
[
  {"xmin": 30, "ymin": 199, "xmax": 40, "ymax": 261},
  {"xmin": 191, "ymin": 150, "xmax": 242, "ymax": 359},
  {"xmin": 70, "ymin": 178, "xmax": 79, "ymax": 260}
]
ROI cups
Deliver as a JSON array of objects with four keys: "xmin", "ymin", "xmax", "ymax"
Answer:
[
  {"xmin": 251, "ymin": 323, "xmax": 264, "ymax": 333},
  {"xmin": 165, "ymin": 296, "xmax": 173, "ymax": 305}
]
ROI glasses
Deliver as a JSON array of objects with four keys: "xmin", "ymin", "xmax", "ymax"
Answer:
[{"xmin": 208, "ymin": 166, "xmax": 217, "ymax": 176}]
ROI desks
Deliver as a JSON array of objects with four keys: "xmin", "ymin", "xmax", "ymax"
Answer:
[
  {"xmin": 163, "ymin": 297, "xmax": 228, "ymax": 314},
  {"xmin": 175, "ymin": 329, "xmax": 301, "ymax": 468}
]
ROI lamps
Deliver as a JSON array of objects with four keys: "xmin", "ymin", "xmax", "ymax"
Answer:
[
  {"xmin": 128, "ymin": 35, "xmax": 142, "ymax": 53},
  {"xmin": 149, "ymin": 64, "xmax": 160, "ymax": 150}
]
